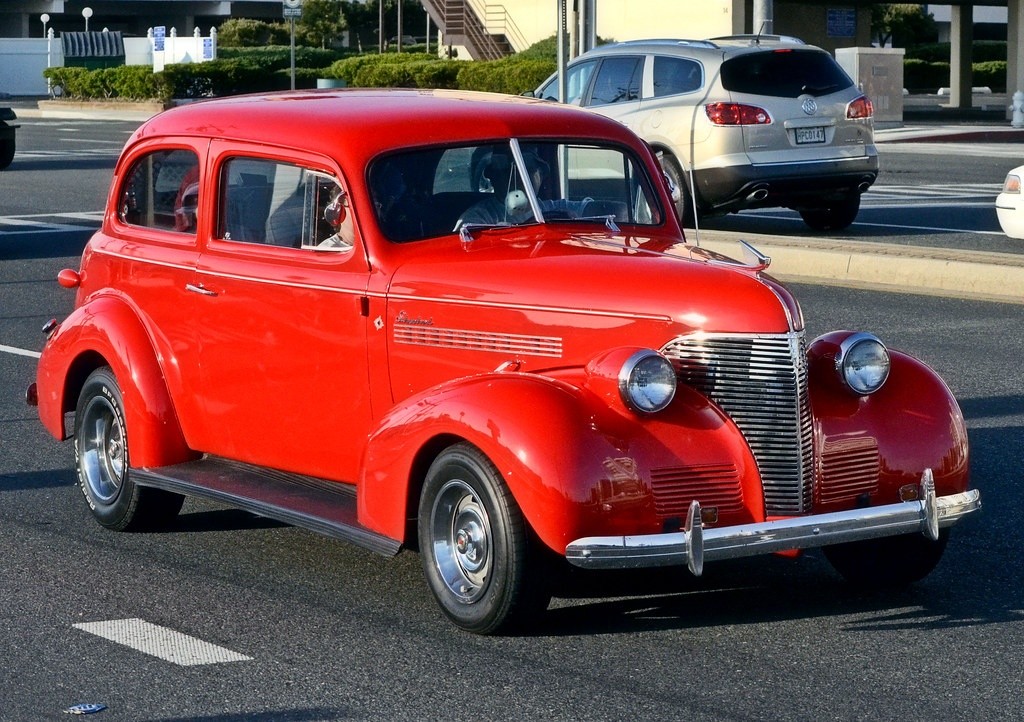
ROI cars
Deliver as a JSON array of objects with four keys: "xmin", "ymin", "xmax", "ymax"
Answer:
[{"xmin": 25, "ymin": 86, "xmax": 985, "ymax": 637}]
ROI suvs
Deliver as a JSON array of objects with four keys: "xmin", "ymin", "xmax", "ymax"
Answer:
[{"xmin": 470, "ymin": 32, "xmax": 881, "ymax": 236}]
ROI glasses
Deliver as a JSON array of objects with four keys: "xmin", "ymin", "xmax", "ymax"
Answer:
[
  {"xmin": 489, "ymin": 159, "xmax": 538, "ymax": 178},
  {"xmin": 343, "ymin": 202, "xmax": 382, "ymax": 210}
]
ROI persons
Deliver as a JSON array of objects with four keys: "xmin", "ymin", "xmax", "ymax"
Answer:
[
  {"xmin": 318, "ymin": 184, "xmax": 382, "ymax": 249},
  {"xmin": 453, "ymin": 142, "xmax": 634, "ymax": 231}
]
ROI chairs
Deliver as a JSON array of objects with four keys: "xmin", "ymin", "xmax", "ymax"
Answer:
[{"xmin": 265, "ymin": 173, "xmax": 341, "ymax": 250}]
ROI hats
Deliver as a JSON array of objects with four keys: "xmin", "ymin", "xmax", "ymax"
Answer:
[{"xmin": 484, "ymin": 142, "xmax": 550, "ymax": 180}]
